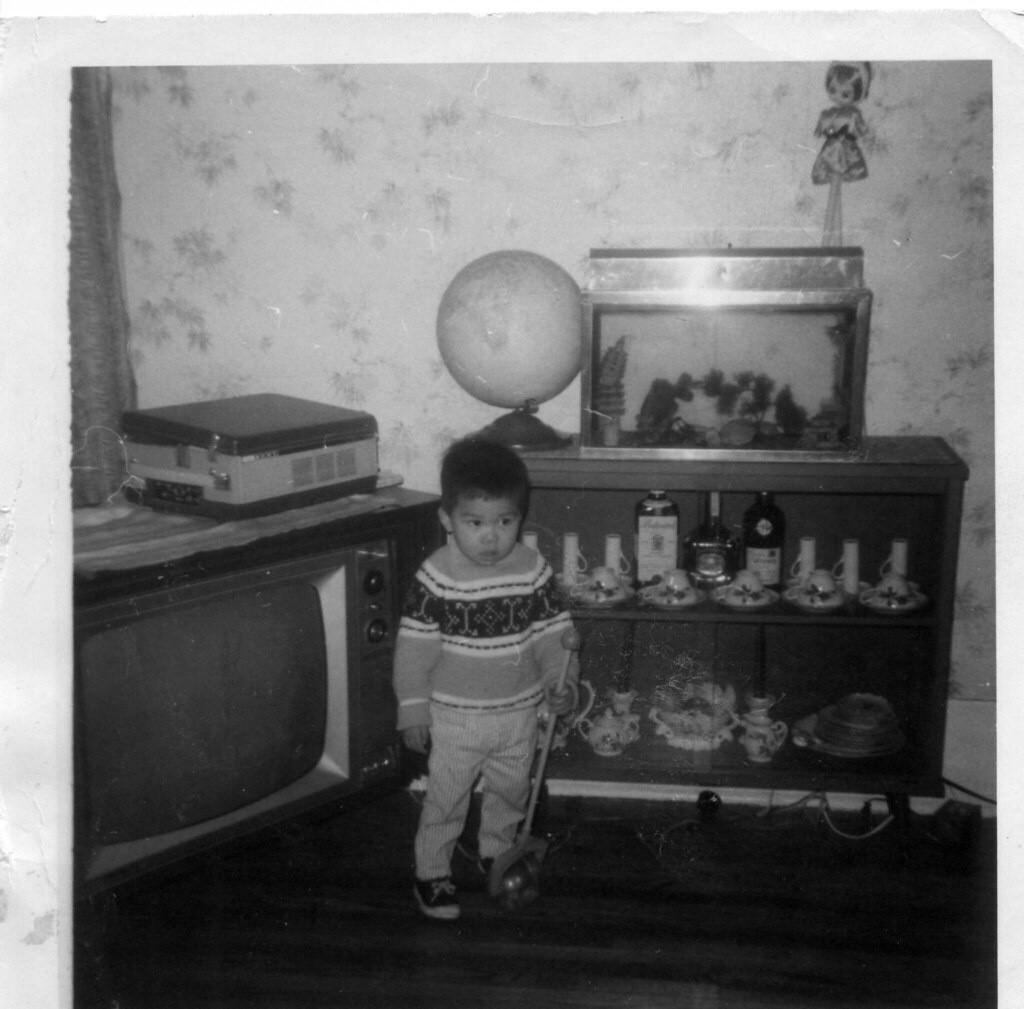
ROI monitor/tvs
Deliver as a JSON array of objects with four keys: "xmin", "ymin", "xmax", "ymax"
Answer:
[{"xmin": 75, "ymin": 519, "xmax": 450, "ymax": 894}]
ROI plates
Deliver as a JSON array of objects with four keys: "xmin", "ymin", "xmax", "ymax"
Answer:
[
  {"xmin": 636, "ymin": 584, "xmax": 706, "ymax": 611},
  {"xmin": 709, "ymin": 585, "xmax": 780, "ymax": 612},
  {"xmin": 856, "ymin": 592, "xmax": 929, "ymax": 615},
  {"xmin": 781, "ymin": 585, "xmax": 853, "ymax": 613},
  {"xmin": 564, "ymin": 582, "xmax": 635, "ymax": 610}
]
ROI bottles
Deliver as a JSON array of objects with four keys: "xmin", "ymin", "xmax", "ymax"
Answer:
[
  {"xmin": 684, "ymin": 489, "xmax": 738, "ymax": 590},
  {"xmin": 741, "ymin": 491, "xmax": 786, "ymax": 591},
  {"xmin": 634, "ymin": 489, "xmax": 681, "ymax": 586}
]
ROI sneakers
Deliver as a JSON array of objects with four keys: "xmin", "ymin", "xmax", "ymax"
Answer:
[
  {"xmin": 478, "ymin": 852, "xmax": 526, "ymax": 882},
  {"xmin": 413, "ymin": 876, "xmax": 460, "ymax": 920}
]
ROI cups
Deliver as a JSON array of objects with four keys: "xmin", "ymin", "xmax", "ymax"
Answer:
[
  {"xmin": 859, "ymin": 574, "xmax": 917, "ymax": 608},
  {"xmin": 713, "ymin": 569, "xmax": 769, "ymax": 604},
  {"xmin": 577, "ymin": 708, "xmax": 640, "ymax": 755},
  {"xmin": 785, "ymin": 568, "xmax": 843, "ymax": 605},
  {"xmin": 743, "ymin": 713, "xmax": 788, "ymax": 763},
  {"xmin": 569, "ymin": 566, "xmax": 627, "ymax": 600},
  {"xmin": 642, "ymin": 569, "xmax": 696, "ymax": 604}
]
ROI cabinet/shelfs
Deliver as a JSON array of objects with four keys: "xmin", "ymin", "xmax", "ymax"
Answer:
[{"xmin": 513, "ymin": 434, "xmax": 969, "ymax": 814}]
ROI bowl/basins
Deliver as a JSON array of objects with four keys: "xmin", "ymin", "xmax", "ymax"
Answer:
[
  {"xmin": 791, "ymin": 713, "xmax": 907, "ymax": 770},
  {"xmin": 813, "ymin": 691, "xmax": 902, "ymax": 751}
]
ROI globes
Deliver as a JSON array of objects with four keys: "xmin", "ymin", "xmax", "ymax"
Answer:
[{"xmin": 435, "ymin": 250, "xmax": 582, "ymax": 451}]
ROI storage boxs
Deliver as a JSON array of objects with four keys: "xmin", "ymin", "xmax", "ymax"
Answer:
[{"xmin": 581, "ymin": 246, "xmax": 873, "ymax": 463}]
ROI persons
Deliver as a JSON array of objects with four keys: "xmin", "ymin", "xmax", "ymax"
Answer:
[
  {"xmin": 393, "ymin": 440, "xmax": 575, "ymax": 919},
  {"xmin": 809, "ymin": 61, "xmax": 876, "ymax": 248}
]
